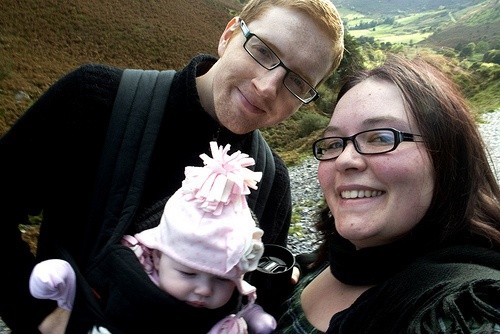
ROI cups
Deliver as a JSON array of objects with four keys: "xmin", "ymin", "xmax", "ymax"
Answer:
[{"xmin": 242, "ymin": 243, "xmax": 295, "ymax": 313}]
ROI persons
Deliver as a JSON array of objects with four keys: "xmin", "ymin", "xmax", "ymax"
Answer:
[
  {"xmin": 30, "ymin": 139, "xmax": 277, "ymax": 334},
  {"xmin": 257, "ymin": 55, "xmax": 500, "ymax": 334},
  {"xmin": 0, "ymin": 0, "xmax": 346, "ymax": 334}
]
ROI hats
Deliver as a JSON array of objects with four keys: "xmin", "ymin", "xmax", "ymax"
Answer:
[{"xmin": 135, "ymin": 141, "xmax": 265, "ymax": 306}]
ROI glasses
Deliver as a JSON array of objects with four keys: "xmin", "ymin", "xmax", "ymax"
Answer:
[
  {"xmin": 239, "ymin": 18, "xmax": 320, "ymax": 105},
  {"xmin": 312, "ymin": 128, "xmax": 430, "ymax": 161}
]
